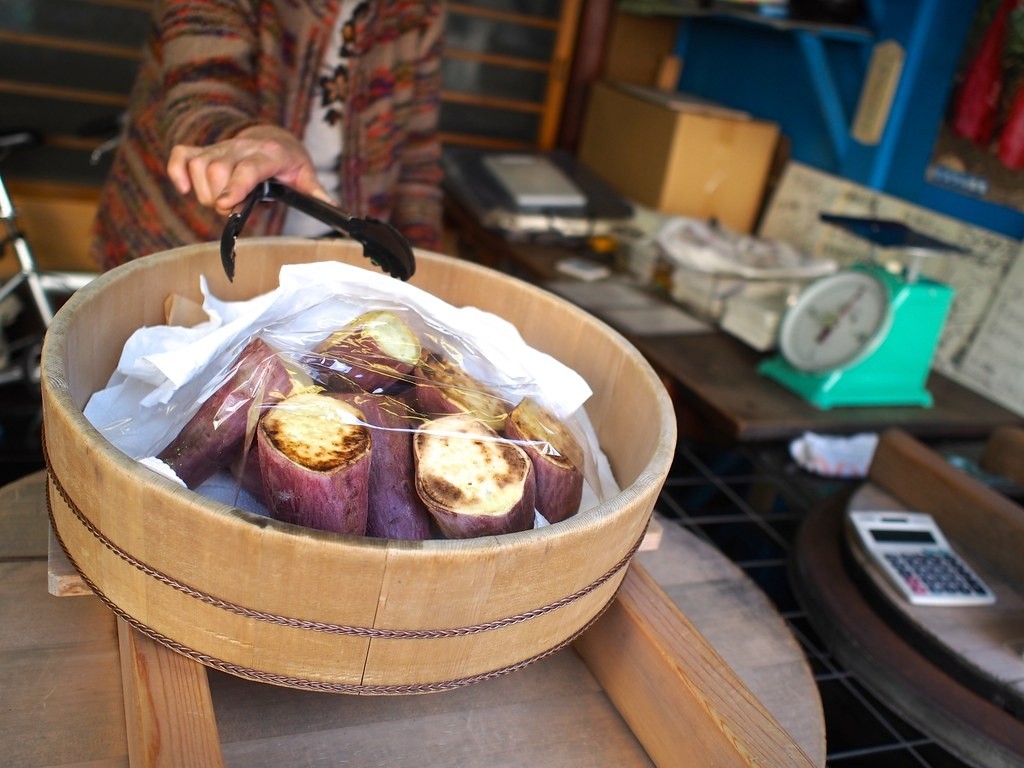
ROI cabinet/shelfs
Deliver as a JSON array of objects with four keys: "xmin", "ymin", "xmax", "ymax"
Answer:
[{"xmin": 609, "ymin": 0, "xmax": 967, "ymax": 198}]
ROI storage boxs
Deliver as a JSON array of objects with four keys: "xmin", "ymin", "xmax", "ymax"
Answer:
[{"xmin": 576, "ymin": 79, "xmax": 777, "ymax": 234}]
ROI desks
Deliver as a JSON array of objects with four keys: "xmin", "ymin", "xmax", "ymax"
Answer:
[{"xmin": 444, "ymin": 202, "xmax": 1024, "ymax": 563}]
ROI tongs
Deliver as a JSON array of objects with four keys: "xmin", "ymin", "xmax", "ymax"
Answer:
[{"xmin": 220, "ymin": 177, "xmax": 416, "ymax": 283}]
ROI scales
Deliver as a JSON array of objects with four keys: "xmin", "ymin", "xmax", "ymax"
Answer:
[{"xmin": 756, "ymin": 210, "xmax": 984, "ymax": 412}]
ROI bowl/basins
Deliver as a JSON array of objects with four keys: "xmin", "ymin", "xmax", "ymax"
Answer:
[{"xmin": 37, "ymin": 238, "xmax": 675, "ymax": 695}]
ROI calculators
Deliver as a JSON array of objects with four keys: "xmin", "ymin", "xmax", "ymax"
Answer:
[{"xmin": 846, "ymin": 508, "xmax": 996, "ymax": 606}]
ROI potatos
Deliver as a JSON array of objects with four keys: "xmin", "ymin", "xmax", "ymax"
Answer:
[{"xmin": 155, "ymin": 308, "xmax": 585, "ymax": 541}]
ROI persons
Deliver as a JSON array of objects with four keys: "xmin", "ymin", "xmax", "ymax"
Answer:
[{"xmin": 91, "ymin": 0, "xmax": 449, "ymax": 272}]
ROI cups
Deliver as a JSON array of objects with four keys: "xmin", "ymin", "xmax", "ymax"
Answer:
[{"xmin": 654, "ymin": 55, "xmax": 682, "ymax": 93}]
